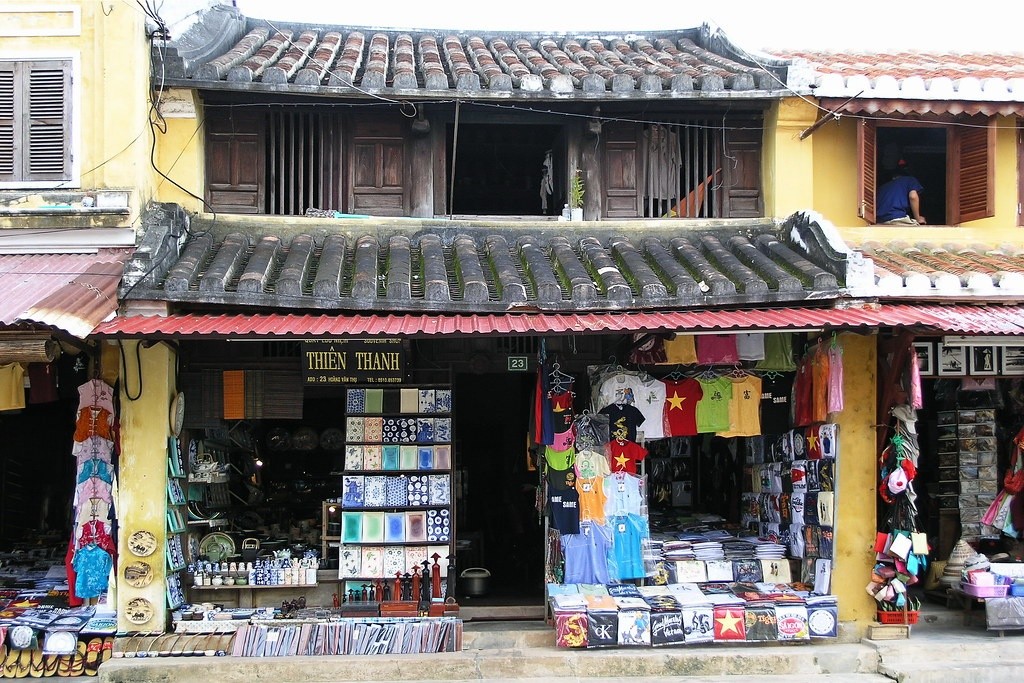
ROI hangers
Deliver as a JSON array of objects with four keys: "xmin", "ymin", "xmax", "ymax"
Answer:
[
  {"xmin": 800, "ymin": 344, "xmax": 809, "ymax": 361},
  {"xmin": 830, "ymin": 332, "xmax": 840, "ymax": 352},
  {"xmin": 92, "ymin": 368, "xmax": 103, "ymax": 385},
  {"xmin": 89, "ymin": 393, "xmax": 104, "ymax": 412},
  {"xmin": 545, "ymin": 361, "xmax": 576, "ymax": 400},
  {"xmin": 89, "ymin": 487, "xmax": 102, "ymax": 501},
  {"xmin": 90, "ymin": 430, "xmax": 99, "ymax": 440},
  {"xmin": 90, "ymin": 450, "xmax": 102, "ymax": 462},
  {"xmin": 812, "ymin": 336, "xmax": 823, "ymax": 355},
  {"xmin": 575, "ymin": 409, "xmax": 630, "ymax": 530},
  {"xmin": 602, "ymin": 354, "xmax": 786, "ymax": 381}
]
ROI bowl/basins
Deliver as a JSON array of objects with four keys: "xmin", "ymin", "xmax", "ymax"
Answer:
[{"xmin": 170, "ymin": 392, "xmax": 184, "ymax": 437}]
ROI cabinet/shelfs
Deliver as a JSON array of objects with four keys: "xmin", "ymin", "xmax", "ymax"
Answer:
[{"xmin": 320, "ymin": 501, "xmax": 342, "ymax": 563}]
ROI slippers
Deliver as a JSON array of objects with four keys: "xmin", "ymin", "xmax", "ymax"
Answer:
[{"xmin": 0, "ymin": 636, "xmax": 113, "ymax": 678}]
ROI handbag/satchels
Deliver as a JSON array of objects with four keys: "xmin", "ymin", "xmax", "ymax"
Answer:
[
  {"xmin": 864, "ymin": 518, "xmax": 930, "ymax": 607},
  {"xmin": 980, "ymin": 445, "xmax": 1024, "ymax": 538}
]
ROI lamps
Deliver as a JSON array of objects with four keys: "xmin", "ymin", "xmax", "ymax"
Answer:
[{"xmin": 943, "ymin": 334, "xmax": 1024, "ymax": 349}]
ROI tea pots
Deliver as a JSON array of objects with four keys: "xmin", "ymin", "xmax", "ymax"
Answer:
[
  {"xmin": 254, "ymin": 549, "xmax": 320, "ymax": 570},
  {"xmin": 192, "ymin": 454, "xmax": 230, "ymax": 474}
]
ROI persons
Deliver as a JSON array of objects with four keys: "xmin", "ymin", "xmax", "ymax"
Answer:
[{"xmin": 877, "ymin": 169, "xmax": 926, "ymax": 225}]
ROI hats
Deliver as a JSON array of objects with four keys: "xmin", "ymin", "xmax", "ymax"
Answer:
[
  {"xmin": 878, "ymin": 405, "xmax": 919, "ymax": 516},
  {"xmin": 939, "ymin": 539, "xmax": 980, "ymax": 588}
]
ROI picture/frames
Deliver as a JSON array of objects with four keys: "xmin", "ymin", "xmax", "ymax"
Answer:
[
  {"xmin": 937, "ymin": 343, "xmax": 967, "ymax": 376},
  {"xmin": 968, "ymin": 346, "xmax": 998, "ymax": 376},
  {"xmin": 910, "ymin": 342, "xmax": 934, "ymax": 377},
  {"xmin": 1001, "ymin": 346, "xmax": 1024, "ymax": 375}
]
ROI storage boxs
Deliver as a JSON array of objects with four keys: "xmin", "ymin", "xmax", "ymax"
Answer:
[
  {"xmin": 877, "ymin": 609, "xmax": 919, "ymax": 625},
  {"xmin": 1010, "ymin": 583, "xmax": 1024, "ymax": 597},
  {"xmin": 962, "ymin": 580, "xmax": 1010, "ymax": 597},
  {"xmin": 867, "ymin": 624, "xmax": 912, "ymax": 640}
]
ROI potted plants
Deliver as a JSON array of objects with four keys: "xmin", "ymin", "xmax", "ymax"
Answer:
[{"xmin": 567, "ymin": 176, "xmax": 586, "ymax": 221}]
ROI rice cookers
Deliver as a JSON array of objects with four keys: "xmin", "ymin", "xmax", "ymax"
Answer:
[{"xmin": 458, "ymin": 567, "xmax": 494, "ymax": 598}]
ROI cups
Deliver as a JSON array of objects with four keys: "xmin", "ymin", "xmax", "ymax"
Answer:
[{"xmin": 182, "ymin": 604, "xmax": 204, "ymax": 619}]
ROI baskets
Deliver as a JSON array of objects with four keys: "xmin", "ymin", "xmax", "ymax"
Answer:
[
  {"xmin": 1011, "ymin": 584, "xmax": 1024, "ymax": 597},
  {"xmin": 878, "ymin": 610, "xmax": 918, "ymax": 624},
  {"xmin": 961, "ymin": 580, "xmax": 1007, "ymax": 598}
]
